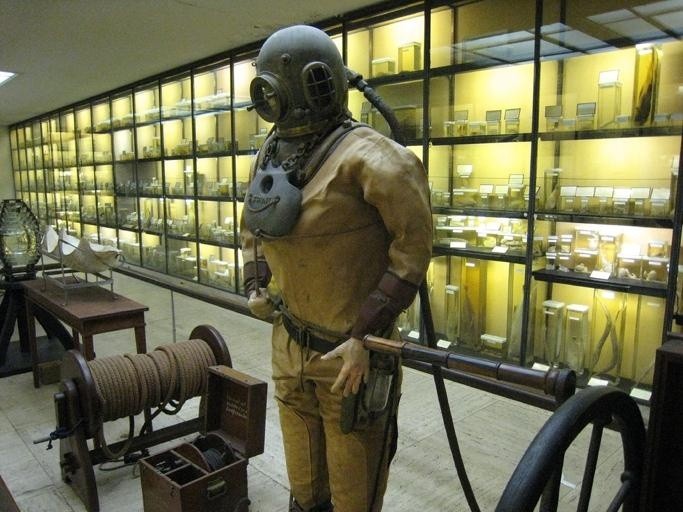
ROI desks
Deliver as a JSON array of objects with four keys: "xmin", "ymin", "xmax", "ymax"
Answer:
[{"xmin": 20, "ymin": 275, "xmax": 150, "ymax": 389}]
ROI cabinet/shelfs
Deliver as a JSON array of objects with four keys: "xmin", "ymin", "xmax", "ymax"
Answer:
[{"xmin": 7, "ymin": 1, "xmax": 683, "ymax": 436}]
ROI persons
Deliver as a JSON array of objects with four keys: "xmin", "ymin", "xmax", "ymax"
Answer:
[{"xmin": 239, "ymin": 23, "xmax": 434, "ymax": 512}]
